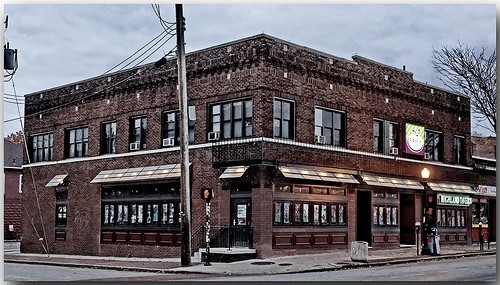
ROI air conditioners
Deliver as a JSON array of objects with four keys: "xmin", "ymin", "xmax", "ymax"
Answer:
[
  {"xmin": 130, "ymin": 142, "xmax": 140, "ymax": 151},
  {"xmin": 389, "ymin": 148, "xmax": 398, "ymax": 155},
  {"xmin": 208, "ymin": 132, "xmax": 219, "ymax": 141},
  {"xmin": 163, "ymin": 139, "xmax": 174, "ymax": 146},
  {"xmin": 424, "ymin": 153, "xmax": 429, "ymax": 160},
  {"xmin": 315, "ymin": 136, "xmax": 326, "ymax": 145}
]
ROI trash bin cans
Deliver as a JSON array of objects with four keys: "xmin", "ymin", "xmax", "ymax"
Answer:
[{"xmin": 427, "ymin": 227, "xmax": 441, "ymax": 256}]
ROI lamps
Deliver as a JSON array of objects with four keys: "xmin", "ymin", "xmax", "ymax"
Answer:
[
  {"xmin": 415, "ymin": 221, "xmax": 421, "ymax": 229},
  {"xmin": 421, "ymin": 168, "xmax": 430, "ymax": 179},
  {"xmin": 479, "ymin": 222, "xmax": 483, "ymax": 226}
]
